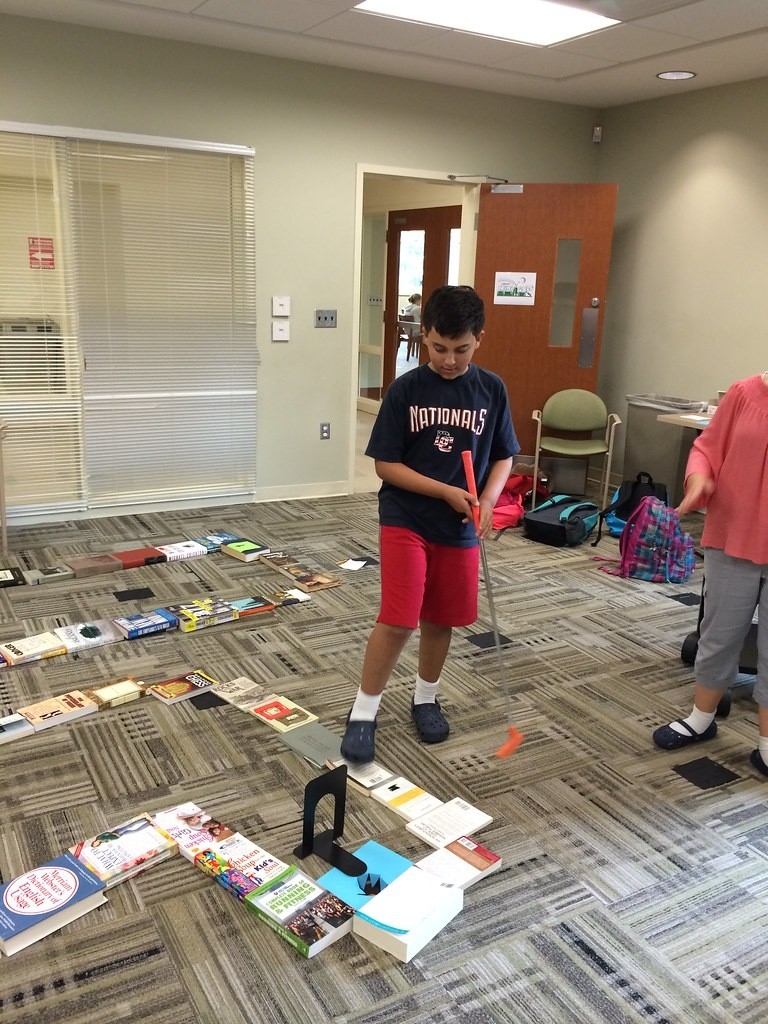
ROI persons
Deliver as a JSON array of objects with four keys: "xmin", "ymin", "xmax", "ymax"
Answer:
[
  {"xmin": 652, "ymin": 371, "xmax": 768, "ymax": 778},
  {"xmin": 187, "ymin": 817, "xmax": 202, "ymax": 831},
  {"xmin": 203, "ymin": 819, "xmax": 220, "ymax": 829},
  {"xmin": 341, "ymin": 285, "xmax": 521, "ymax": 764},
  {"xmin": 403, "ymin": 293, "xmax": 421, "ymax": 337},
  {"xmin": 209, "ymin": 824, "xmax": 224, "ymax": 836}
]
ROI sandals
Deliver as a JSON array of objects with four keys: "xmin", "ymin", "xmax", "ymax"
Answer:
[
  {"xmin": 750, "ymin": 749, "xmax": 768, "ymax": 777},
  {"xmin": 410, "ymin": 693, "xmax": 450, "ymax": 744},
  {"xmin": 340, "ymin": 707, "xmax": 378, "ymax": 764},
  {"xmin": 652, "ymin": 718, "xmax": 718, "ymax": 751}
]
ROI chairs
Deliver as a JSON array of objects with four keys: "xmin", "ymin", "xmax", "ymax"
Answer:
[
  {"xmin": 398, "ymin": 315, "xmax": 421, "ymax": 362},
  {"xmin": 531, "ymin": 389, "xmax": 623, "ymax": 522}
]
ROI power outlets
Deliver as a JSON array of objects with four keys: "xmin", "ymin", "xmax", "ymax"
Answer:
[{"xmin": 320, "ymin": 422, "xmax": 332, "ymax": 440}]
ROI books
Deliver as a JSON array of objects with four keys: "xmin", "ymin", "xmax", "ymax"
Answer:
[
  {"xmin": 0, "ymin": 532, "xmax": 342, "ymax": 668},
  {"xmin": 0, "ymin": 667, "xmax": 503, "ymax": 964}
]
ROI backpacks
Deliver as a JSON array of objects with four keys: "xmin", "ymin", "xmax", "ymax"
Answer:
[
  {"xmin": 524, "ymin": 493, "xmax": 600, "ymax": 547},
  {"xmin": 591, "ymin": 471, "xmax": 670, "ymax": 547},
  {"xmin": 593, "ymin": 496, "xmax": 696, "ymax": 584}
]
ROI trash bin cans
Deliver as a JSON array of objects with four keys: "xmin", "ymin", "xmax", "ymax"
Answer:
[{"xmin": 621, "ymin": 392, "xmax": 709, "ymax": 509}]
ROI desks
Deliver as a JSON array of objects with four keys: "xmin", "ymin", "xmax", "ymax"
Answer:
[{"xmin": 657, "ymin": 412, "xmax": 714, "ymax": 436}]
